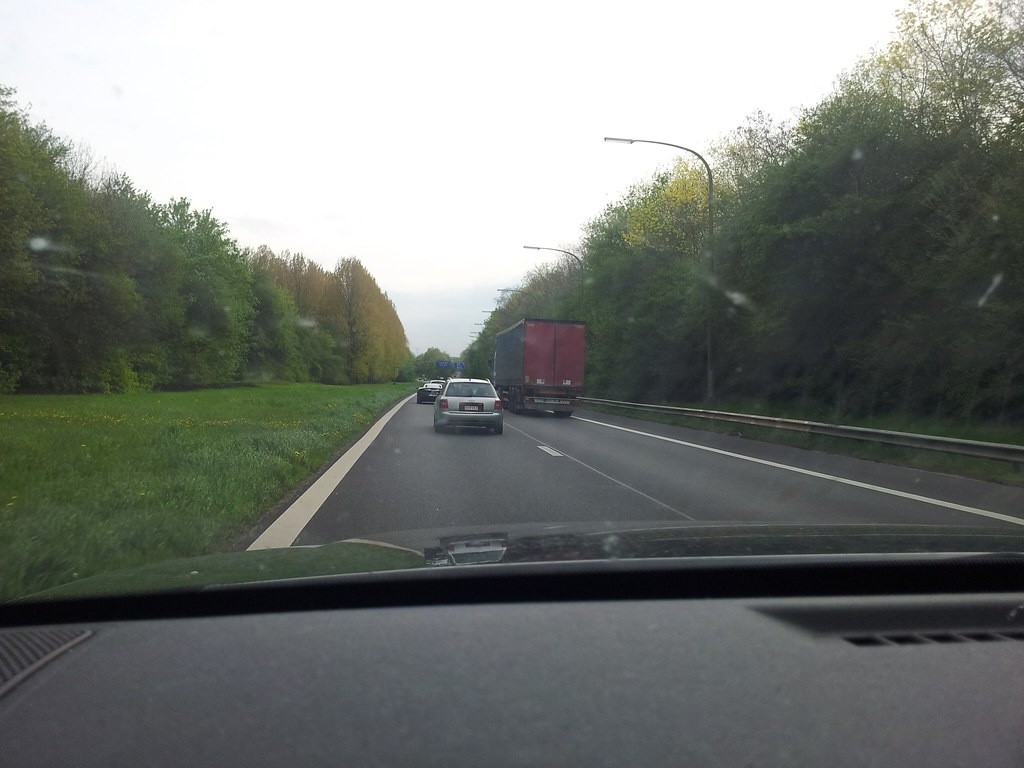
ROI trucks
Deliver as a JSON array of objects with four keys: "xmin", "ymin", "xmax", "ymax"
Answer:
[{"xmin": 489, "ymin": 318, "xmax": 589, "ymax": 417}]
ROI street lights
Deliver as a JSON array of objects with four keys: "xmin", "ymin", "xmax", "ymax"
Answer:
[
  {"xmin": 604, "ymin": 137, "xmax": 715, "ymax": 400},
  {"xmin": 523, "ymin": 246, "xmax": 584, "ymax": 315},
  {"xmin": 497, "ymin": 289, "xmax": 539, "ymax": 313}
]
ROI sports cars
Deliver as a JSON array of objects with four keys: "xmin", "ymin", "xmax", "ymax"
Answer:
[{"xmin": 416, "ymin": 383, "xmax": 442, "ymax": 404}]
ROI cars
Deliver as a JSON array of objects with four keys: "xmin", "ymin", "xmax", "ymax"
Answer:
[
  {"xmin": 433, "ymin": 377, "xmax": 505, "ymax": 434},
  {"xmin": 431, "ymin": 380, "xmax": 446, "ymax": 386}
]
